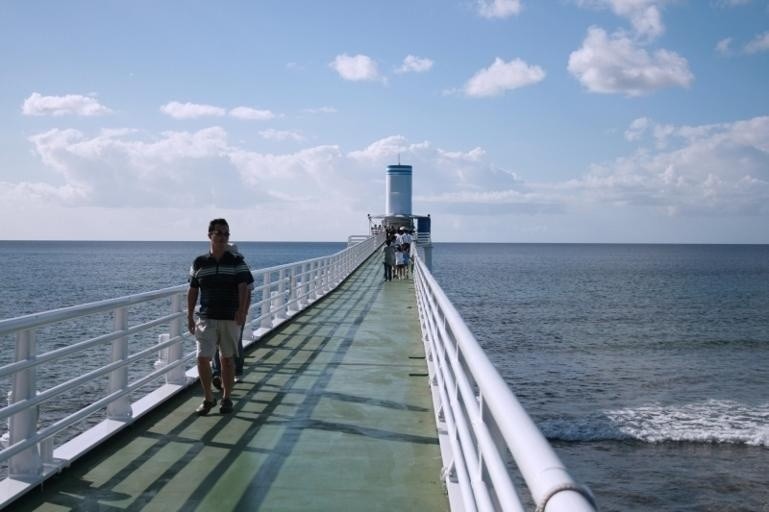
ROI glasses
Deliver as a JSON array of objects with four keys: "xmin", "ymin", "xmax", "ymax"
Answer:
[{"xmin": 212, "ymin": 230, "xmax": 230, "ymax": 237}]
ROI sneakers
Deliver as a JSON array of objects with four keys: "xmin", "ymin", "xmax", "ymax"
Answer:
[
  {"xmin": 219, "ymin": 399, "xmax": 233, "ymax": 414},
  {"xmin": 195, "ymin": 398, "xmax": 217, "ymax": 416},
  {"xmin": 234, "ymin": 375, "xmax": 240, "ymax": 383},
  {"xmin": 212, "ymin": 376, "xmax": 222, "ymax": 390}
]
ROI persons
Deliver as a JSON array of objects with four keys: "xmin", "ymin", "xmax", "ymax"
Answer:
[
  {"xmin": 187, "ymin": 219, "xmax": 255, "ymax": 415},
  {"xmin": 372, "ymin": 224, "xmax": 412, "ymax": 282},
  {"xmin": 211, "ymin": 242, "xmax": 254, "ymax": 389}
]
